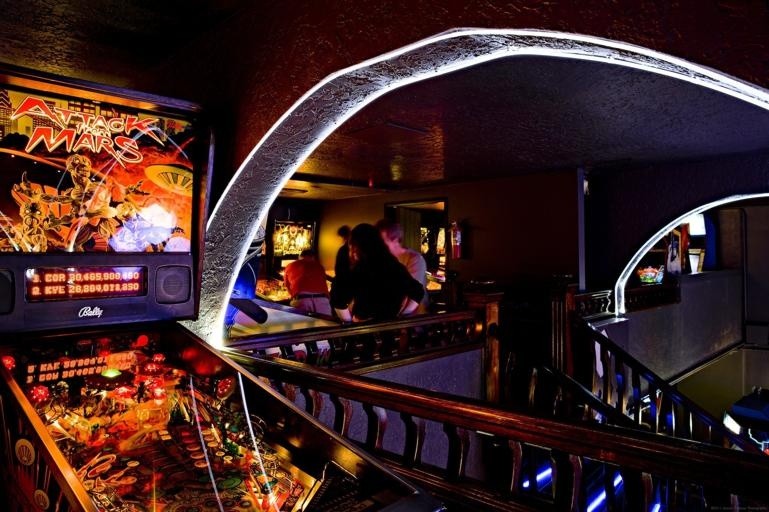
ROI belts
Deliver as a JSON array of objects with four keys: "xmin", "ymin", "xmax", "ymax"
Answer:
[{"xmin": 293, "ymin": 293, "xmax": 327, "ymax": 300}]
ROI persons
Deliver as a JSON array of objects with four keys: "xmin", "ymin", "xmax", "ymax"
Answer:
[{"xmin": 283, "ymin": 221, "xmax": 430, "ymax": 330}]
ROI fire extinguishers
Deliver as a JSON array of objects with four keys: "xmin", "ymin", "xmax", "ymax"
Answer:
[{"xmin": 447, "ymin": 218, "xmax": 462, "ymax": 259}]
